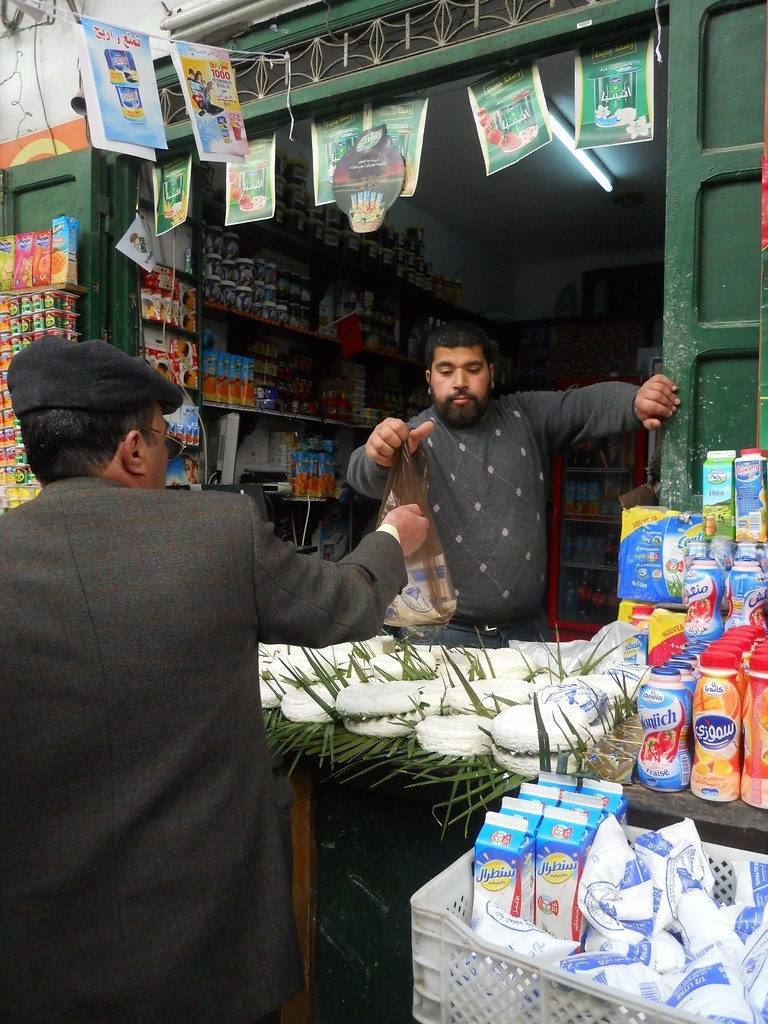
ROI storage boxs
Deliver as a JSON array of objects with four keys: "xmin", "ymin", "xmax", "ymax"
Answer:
[
  {"xmin": 305, "ymin": 453, "xmax": 318, "ymax": 498},
  {"xmin": 0, "ymin": 234, "xmax": 16, "ymax": 292},
  {"xmin": 291, "ymin": 451, "xmax": 308, "ymax": 497},
  {"xmin": 51, "ymin": 215, "xmax": 79, "ymax": 285},
  {"xmin": 408, "ymin": 825, "xmax": 768, "ymax": 1024},
  {"xmin": 201, "ymin": 348, "xmax": 217, "ymax": 401},
  {"xmin": 241, "ymin": 357, "xmax": 256, "ymax": 407},
  {"xmin": 14, "ymin": 231, "xmax": 34, "ymax": 289},
  {"xmin": 216, "ymin": 351, "xmax": 229, "ymax": 404},
  {"xmin": 229, "ymin": 354, "xmax": 244, "ymax": 405},
  {"xmin": 316, "ymin": 453, "xmax": 335, "ymax": 497},
  {"xmin": 32, "ymin": 229, "xmax": 53, "ymax": 287}
]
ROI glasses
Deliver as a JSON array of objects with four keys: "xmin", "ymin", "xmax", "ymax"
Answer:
[{"xmin": 122, "ymin": 426, "xmax": 186, "ymax": 462}]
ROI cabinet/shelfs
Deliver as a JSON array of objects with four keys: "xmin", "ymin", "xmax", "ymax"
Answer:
[
  {"xmin": 507, "ymin": 308, "xmax": 663, "ymax": 512},
  {"xmin": 201, "ymin": 198, "xmax": 530, "ymax": 554}
]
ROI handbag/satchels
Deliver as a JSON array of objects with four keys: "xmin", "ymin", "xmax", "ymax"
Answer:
[{"xmin": 375, "ymin": 427, "xmax": 457, "ymax": 627}]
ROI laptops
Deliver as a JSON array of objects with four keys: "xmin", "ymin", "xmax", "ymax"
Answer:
[{"xmin": 163, "ymin": 483, "xmax": 318, "ymax": 554}]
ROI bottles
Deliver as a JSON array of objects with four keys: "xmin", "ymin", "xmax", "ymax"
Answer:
[
  {"xmin": 561, "ymin": 532, "xmax": 620, "ymax": 624},
  {"xmin": 638, "ymin": 536, "xmax": 768, "ymax": 808}
]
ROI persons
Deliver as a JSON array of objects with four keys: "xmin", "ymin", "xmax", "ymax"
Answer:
[
  {"xmin": 347, "ymin": 326, "xmax": 681, "ymax": 651},
  {"xmin": 187, "ymin": 67, "xmax": 208, "ymax": 106},
  {"xmin": 180, "ymin": 446, "xmax": 205, "ymax": 485},
  {"xmin": 0, "ymin": 333, "xmax": 430, "ymax": 1024}
]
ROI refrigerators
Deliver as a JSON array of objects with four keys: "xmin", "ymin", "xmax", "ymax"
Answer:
[{"xmin": 552, "ymin": 376, "xmax": 646, "ymax": 631}]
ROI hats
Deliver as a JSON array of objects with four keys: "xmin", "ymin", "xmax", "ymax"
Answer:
[{"xmin": 6, "ymin": 335, "xmax": 184, "ymax": 420}]
